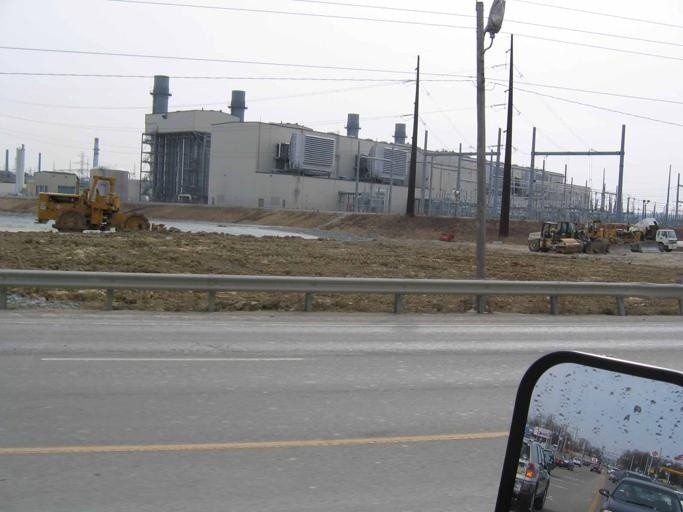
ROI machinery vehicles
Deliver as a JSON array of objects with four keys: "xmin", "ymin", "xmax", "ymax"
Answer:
[
  {"xmin": 528, "ymin": 217, "xmax": 677, "ymax": 254},
  {"xmin": 34, "ymin": 176, "xmax": 150, "ymax": 233}
]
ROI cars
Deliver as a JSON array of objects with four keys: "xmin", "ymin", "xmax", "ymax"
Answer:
[
  {"xmin": 512, "ymin": 436, "xmax": 591, "ymax": 509},
  {"xmin": 590, "ymin": 464, "xmax": 601, "ymax": 474},
  {"xmin": 599, "ymin": 467, "xmax": 683, "ymax": 512}
]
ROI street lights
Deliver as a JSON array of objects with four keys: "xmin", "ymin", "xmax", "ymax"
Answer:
[{"xmin": 475, "ymin": 0, "xmax": 506, "ymax": 312}]
